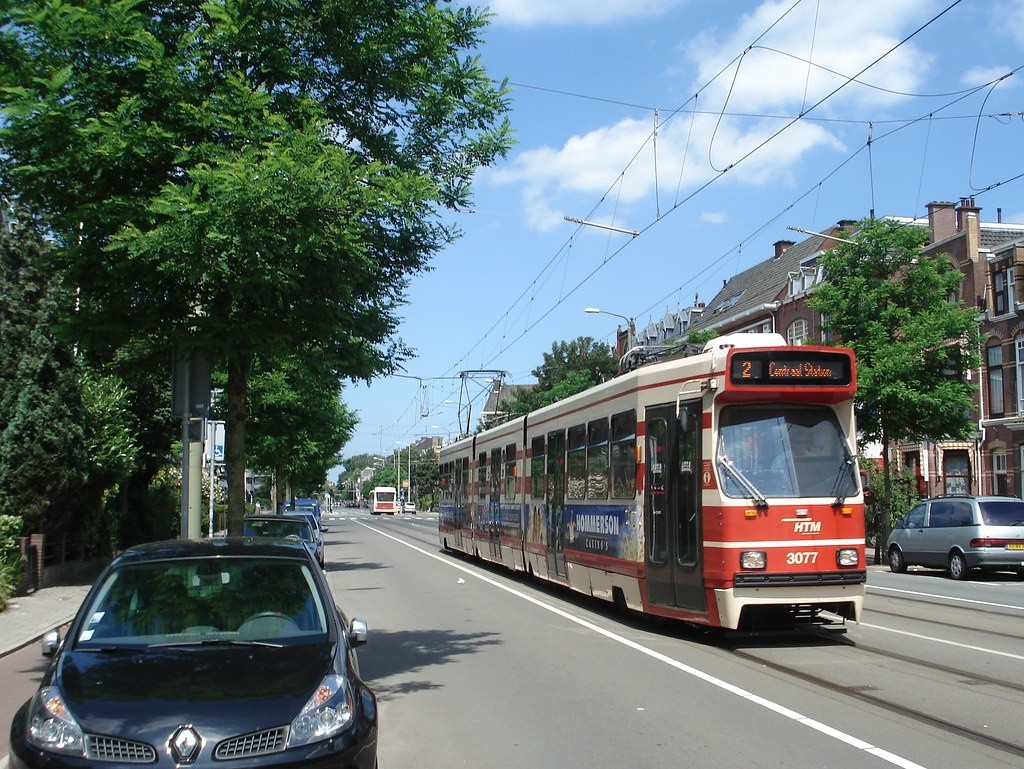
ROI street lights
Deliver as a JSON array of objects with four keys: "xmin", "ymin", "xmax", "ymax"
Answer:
[
  {"xmin": 374, "ymin": 457, "xmax": 384, "ymax": 467},
  {"xmin": 396, "ymin": 441, "xmax": 410, "ymax": 502},
  {"xmin": 383, "ymin": 451, "xmax": 395, "ymax": 468},
  {"xmin": 446, "ymin": 400, "xmax": 468, "ymax": 436},
  {"xmin": 584, "ymin": 307, "xmax": 631, "ymax": 371},
  {"xmin": 365, "ymin": 468, "xmax": 376, "ymax": 477},
  {"xmin": 432, "ymin": 425, "xmax": 450, "ymax": 444}
]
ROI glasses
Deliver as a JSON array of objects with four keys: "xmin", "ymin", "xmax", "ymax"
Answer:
[{"xmin": 792, "ymin": 439, "xmax": 811, "ymax": 445}]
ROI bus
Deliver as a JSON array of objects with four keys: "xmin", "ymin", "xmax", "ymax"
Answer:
[
  {"xmin": 369, "ymin": 486, "xmax": 397, "ymax": 515},
  {"xmin": 437, "ymin": 334, "xmax": 868, "ymax": 632}
]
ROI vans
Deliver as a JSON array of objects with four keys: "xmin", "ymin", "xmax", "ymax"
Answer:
[
  {"xmin": 284, "ymin": 499, "xmax": 322, "ymax": 532},
  {"xmin": 884, "ymin": 493, "xmax": 1024, "ymax": 580}
]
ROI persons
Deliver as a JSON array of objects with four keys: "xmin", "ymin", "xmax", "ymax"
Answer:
[{"xmin": 771, "ymin": 433, "xmax": 816, "ymax": 482}]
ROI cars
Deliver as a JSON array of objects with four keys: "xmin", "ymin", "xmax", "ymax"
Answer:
[
  {"xmin": 259, "ymin": 511, "xmax": 329, "ymax": 569},
  {"xmin": 8, "ymin": 533, "xmax": 379, "ymax": 769},
  {"xmin": 402, "ymin": 502, "xmax": 416, "ymax": 514},
  {"xmin": 243, "ymin": 516, "xmax": 319, "ymax": 561}
]
